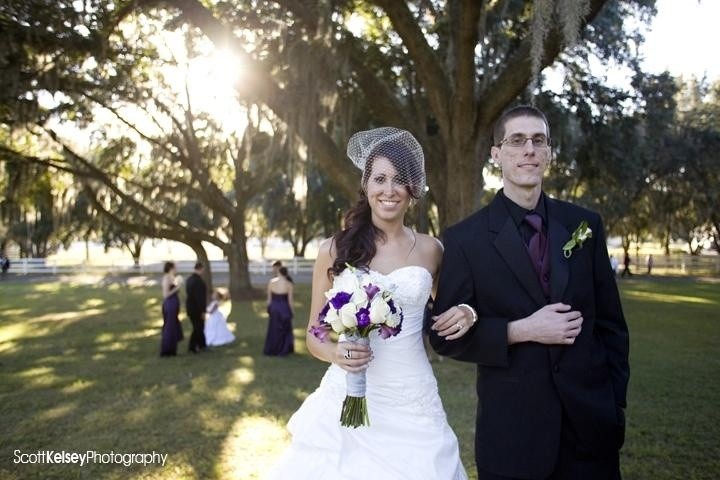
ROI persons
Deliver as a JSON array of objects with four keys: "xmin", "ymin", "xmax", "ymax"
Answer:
[
  {"xmin": 428, "ymin": 107, "xmax": 630, "ymax": 480},
  {"xmin": 265, "ymin": 127, "xmax": 470, "ymax": 479},
  {"xmin": 609, "ymin": 253, "xmax": 617, "ymax": 276},
  {"xmin": 160, "ymin": 262, "xmax": 184, "ymax": 357},
  {"xmin": 203, "ymin": 291, "xmax": 236, "ymax": 347},
  {"xmin": 264, "ymin": 267, "xmax": 296, "ymax": 356},
  {"xmin": 185, "ymin": 262, "xmax": 207, "ymax": 354},
  {"xmin": 271, "ymin": 260, "xmax": 280, "ymax": 277},
  {"xmin": 645, "ymin": 254, "xmax": 653, "ymax": 273},
  {"xmin": 621, "ymin": 253, "xmax": 632, "ymax": 277}
]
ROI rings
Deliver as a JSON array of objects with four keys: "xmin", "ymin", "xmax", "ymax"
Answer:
[
  {"xmin": 344, "ymin": 349, "xmax": 351, "ymax": 359},
  {"xmin": 457, "ymin": 322, "xmax": 463, "ymax": 329}
]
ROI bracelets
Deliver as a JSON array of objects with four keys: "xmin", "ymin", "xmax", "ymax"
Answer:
[{"xmin": 458, "ymin": 304, "xmax": 478, "ymax": 323}]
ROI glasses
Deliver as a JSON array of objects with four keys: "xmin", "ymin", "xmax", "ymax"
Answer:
[{"xmin": 496, "ymin": 135, "xmax": 553, "ymax": 148}]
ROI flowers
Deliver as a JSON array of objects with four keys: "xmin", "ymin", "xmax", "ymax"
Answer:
[{"xmin": 308, "ymin": 282, "xmax": 403, "ymax": 429}]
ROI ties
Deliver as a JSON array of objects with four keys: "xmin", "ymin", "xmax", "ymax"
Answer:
[{"xmin": 524, "ymin": 214, "xmax": 549, "ymax": 297}]
